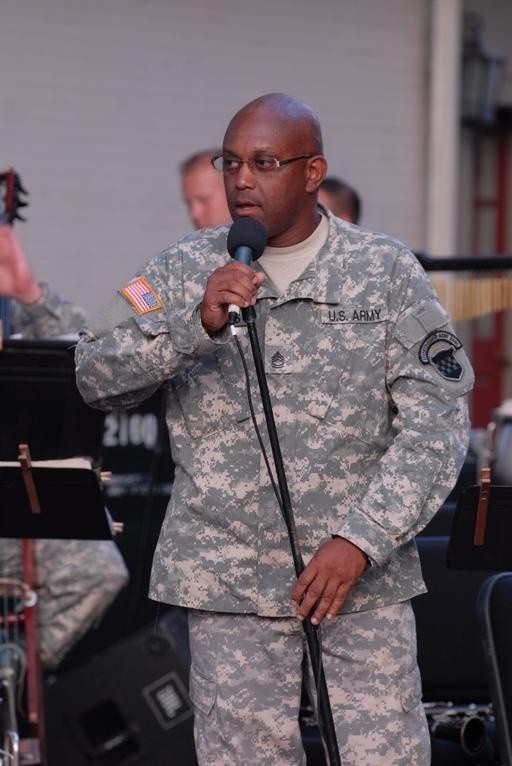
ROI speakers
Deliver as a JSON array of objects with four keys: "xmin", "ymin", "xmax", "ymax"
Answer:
[{"xmin": 42, "ymin": 607, "xmax": 198, "ymax": 766}]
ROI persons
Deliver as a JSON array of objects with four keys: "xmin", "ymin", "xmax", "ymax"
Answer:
[
  {"xmin": 71, "ymin": 92, "xmax": 479, "ymax": 766},
  {"xmin": 178, "ymin": 150, "xmax": 245, "ymax": 228},
  {"xmin": 317, "ymin": 180, "xmax": 366, "ymax": 224},
  {"xmin": 0, "ymin": 168, "xmax": 135, "ymax": 691}
]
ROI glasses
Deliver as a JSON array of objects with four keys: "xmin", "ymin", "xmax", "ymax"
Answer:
[{"xmin": 210, "ymin": 152, "xmax": 314, "ymax": 171}]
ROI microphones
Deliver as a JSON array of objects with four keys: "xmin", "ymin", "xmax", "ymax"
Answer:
[{"xmin": 227, "ymin": 217, "xmax": 267, "ymax": 326}]
